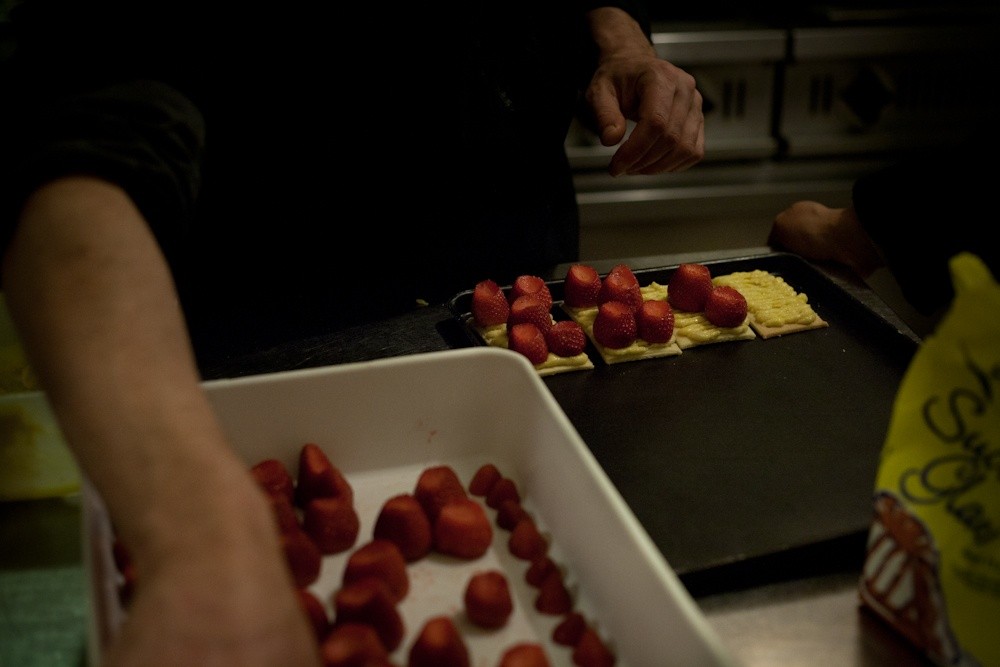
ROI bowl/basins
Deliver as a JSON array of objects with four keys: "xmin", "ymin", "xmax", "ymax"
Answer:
[{"xmin": 78, "ymin": 346, "xmax": 739, "ymax": 667}]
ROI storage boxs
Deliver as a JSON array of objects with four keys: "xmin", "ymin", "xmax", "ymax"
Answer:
[{"xmin": 101, "ymin": 345, "xmax": 743, "ymax": 667}]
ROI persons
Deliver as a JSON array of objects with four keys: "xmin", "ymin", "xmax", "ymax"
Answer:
[
  {"xmin": 771, "ymin": 0, "xmax": 1000, "ymax": 667},
  {"xmin": 0, "ymin": 0, "xmax": 709, "ymax": 667}
]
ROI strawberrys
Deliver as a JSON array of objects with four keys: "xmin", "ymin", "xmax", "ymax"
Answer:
[{"xmin": 114, "ymin": 444, "xmax": 614, "ymax": 667}]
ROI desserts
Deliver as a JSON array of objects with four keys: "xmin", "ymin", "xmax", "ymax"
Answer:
[{"xmin": 464, "ymin": 264, "xmax": 829, "ymax": 377}]
